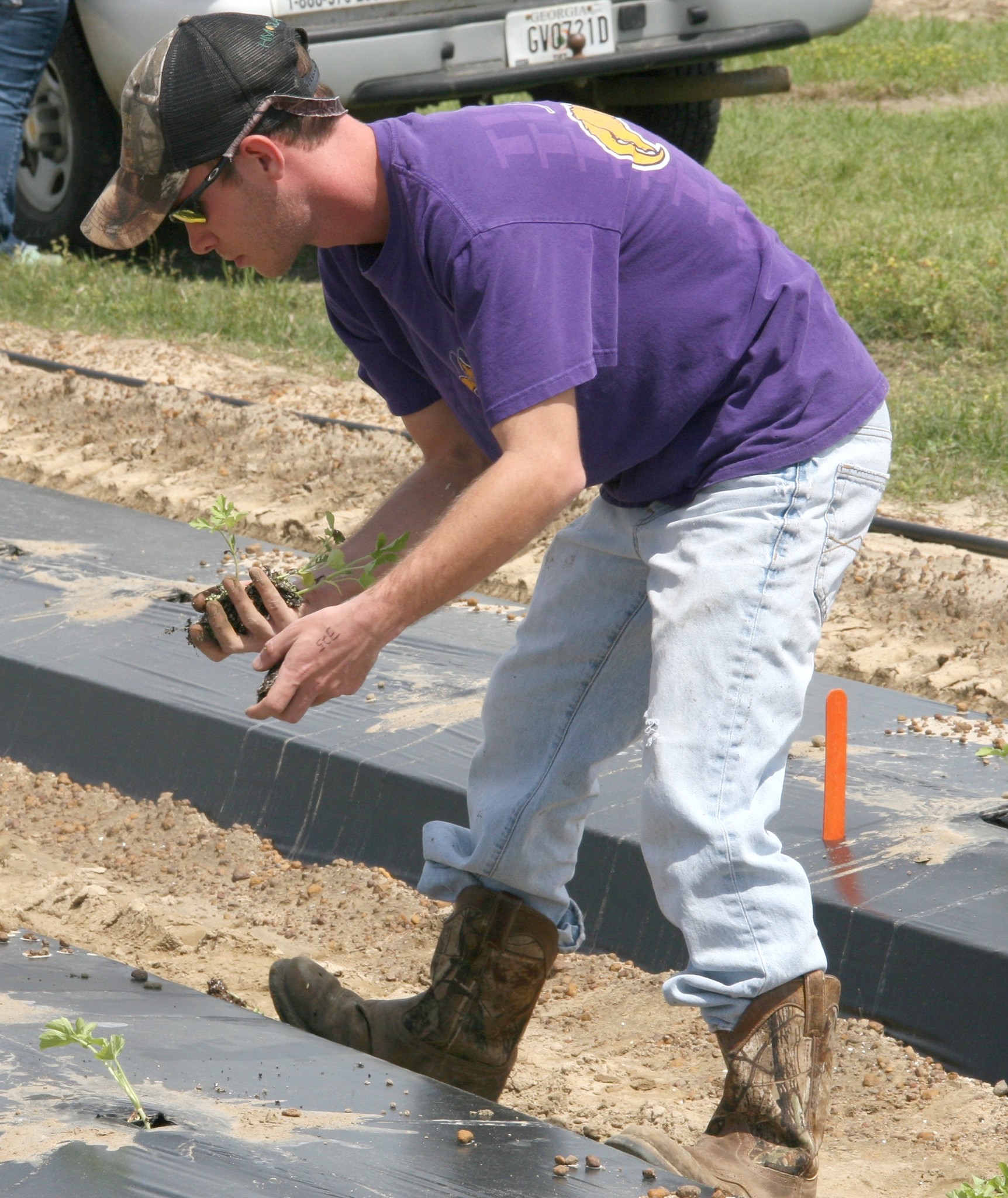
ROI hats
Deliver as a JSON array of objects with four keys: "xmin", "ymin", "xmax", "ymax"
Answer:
[{"xmin": 79, "ymin": 12, "xmax": 319, "ymax": 251}]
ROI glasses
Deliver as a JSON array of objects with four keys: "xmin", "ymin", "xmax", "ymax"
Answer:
[{"xmin": 168, "ymin": 99, "xmax": 274, "ymax": 223}]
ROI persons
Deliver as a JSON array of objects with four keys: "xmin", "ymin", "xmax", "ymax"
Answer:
[
  {"xmin": 84, "ymin": 7, "xmax": 892, "ymax": 1198},
  {"xmin": 0, "ymin": 0, "xmax": 68, "ymax": 256}
]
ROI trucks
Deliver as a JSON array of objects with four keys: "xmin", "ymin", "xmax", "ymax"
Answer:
[{"xmin": 8, "ymin": 2, "xmax": 876, "ymax": 253}]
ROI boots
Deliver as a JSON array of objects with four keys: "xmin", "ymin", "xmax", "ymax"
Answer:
[
  {"xmin": 605, "ymin": 971, "xmax": 841, "ymax": 1198},
  {"xmin": 266, "ymin": 887, "xmax": 558, "ymax": 1105}
]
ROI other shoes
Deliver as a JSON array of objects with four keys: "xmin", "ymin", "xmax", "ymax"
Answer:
[{"xmin": 11, "ymin": 243, "xmax": 63, "ymax": 270}]
